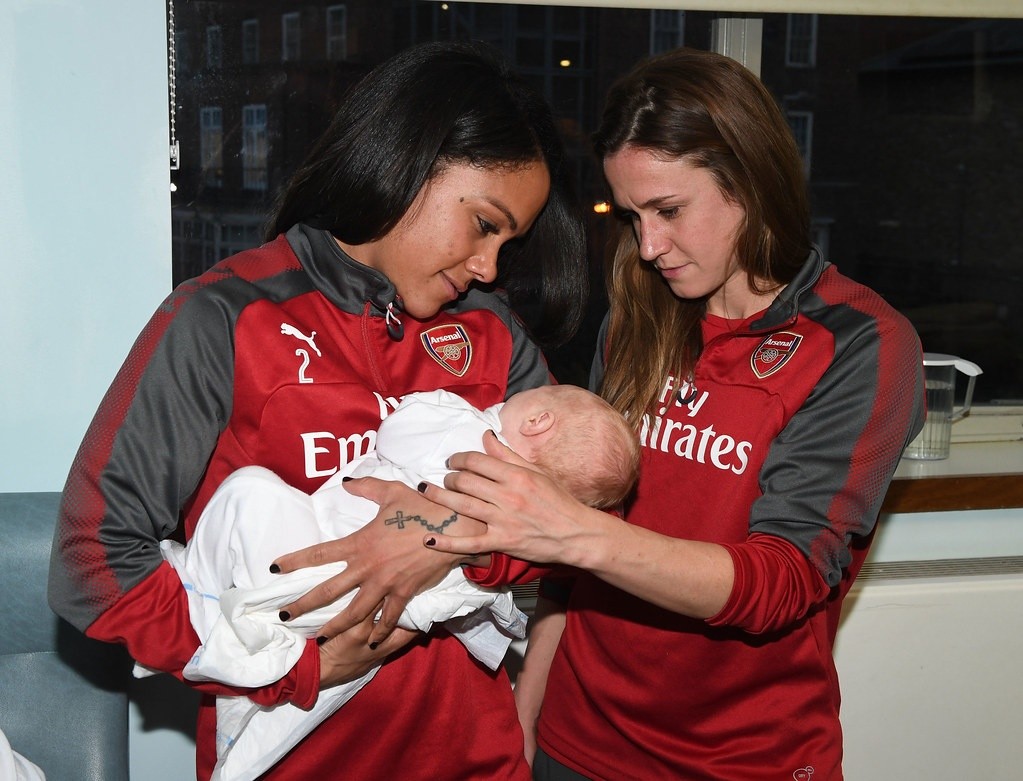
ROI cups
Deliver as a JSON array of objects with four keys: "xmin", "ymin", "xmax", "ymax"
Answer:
[{"xmin": 902, "ymin": 361, "xmax": 977, "ymax": 460}]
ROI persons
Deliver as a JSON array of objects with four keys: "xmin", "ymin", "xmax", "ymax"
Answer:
[
  {"xmin": 132, "ymin": 381, "xmax": 645, "ymax": 682},
  {"xmin": 49, "ymin": 41, "xmax": 564, "ymax": 781},
  {"xmin": 417, "ymin": 48, "xmax": 929, "ymax": 780}
]
